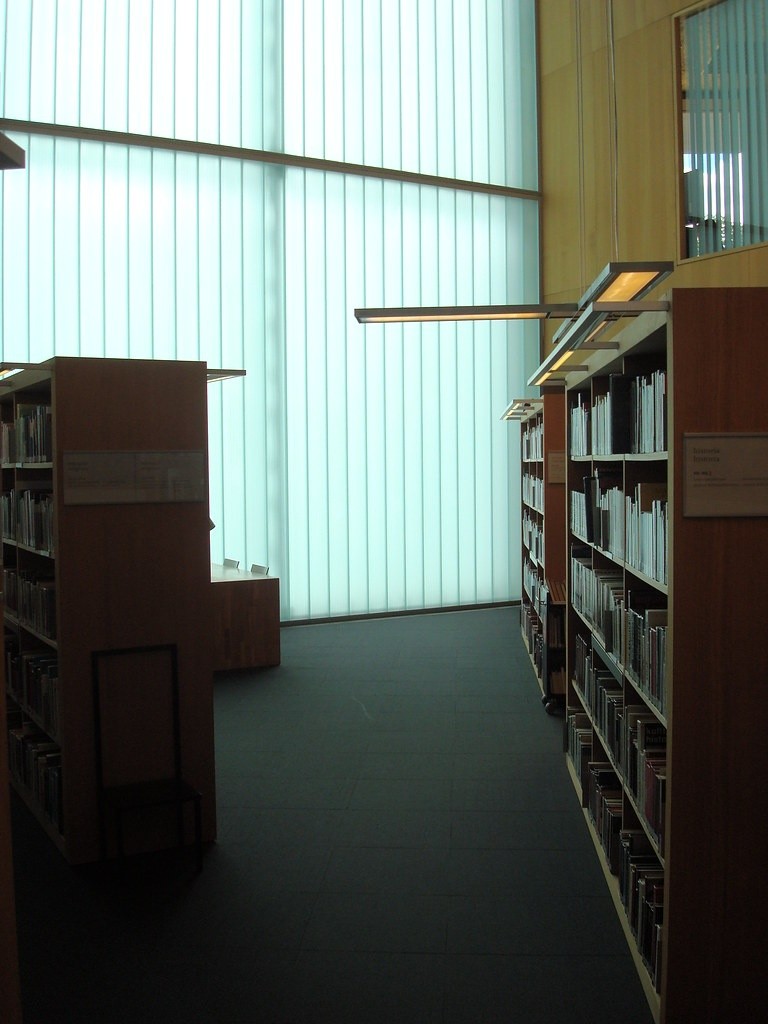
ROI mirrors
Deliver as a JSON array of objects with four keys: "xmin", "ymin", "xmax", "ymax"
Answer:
[{"xmin": 670, "ymin": 0, "xmax": 768, "ymax": 265}]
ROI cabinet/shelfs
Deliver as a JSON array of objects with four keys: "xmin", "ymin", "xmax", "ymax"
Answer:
[
  {"xmin": 500, "ymin": 394, "xmax": 566, "ymax": 715},
  {"xmin": 0, "ymin": 356, "xmax": 248, "ymax": 871},
  {"xmin": 564, "ymin": 287, "xmax": 768, "ymax": 1024}
]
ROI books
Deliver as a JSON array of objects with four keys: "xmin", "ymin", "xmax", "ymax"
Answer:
[
  {"xmin": 522, "ymin": 509, "xmax": 543, "ymax": 563},
  {"xmin": 524, "ymin": 604, "xmax": 544, "ymax": 679},
  {"xmin": 0, "ymin": 568, "xmax": 56, "ymax": 640},
  {"xmin": 522, "ymin": 423, "xmax": 544, "ymax": 460},
  {"xmin": 0, "ymin": 404, "xmax": 52, "ymax": 464},
  {"xmin": 570, "ymin": 557, "xmax": 667, "ymax": 719},
  {"xmin": 568, "ymin": 713, "xmax": 661, "ymax": 994},
  {"xmin": 573, "ymin": 633, "xmax": 666, "ymax": 858},
  {"xmin": 571, "ymin": 369, "xmax": 666, "ymax": 457},
  {"xmin": 524, "ymin": 563, "xmax": 545, "ymax": 622},
  {"xmin": 6, "ymin": 648, "xmax": 57, "ymax": 735},
  {"xmin": 7, "ymin": 733, "xmax": 61, "ymax": 831},
  {"xmin": 570, "ymin": 468, "xmax": 668, "ymax": 585},
  {"xmin": 523, "ymin": 473, "xmax": 545, "ymax": 513},
  {"xmin": 0, "ymin": 488, "xmax": 54, "ymax": 552}
]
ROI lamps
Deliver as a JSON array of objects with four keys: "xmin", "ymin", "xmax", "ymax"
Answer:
[{"xmin": 353, "ymin": 1, "xmax": 674, "ymax": 421}]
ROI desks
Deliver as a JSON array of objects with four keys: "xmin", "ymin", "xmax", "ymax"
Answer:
[{"xmin": 211, "ymin": 563, "xmax": 281, "ymax": 671}]
